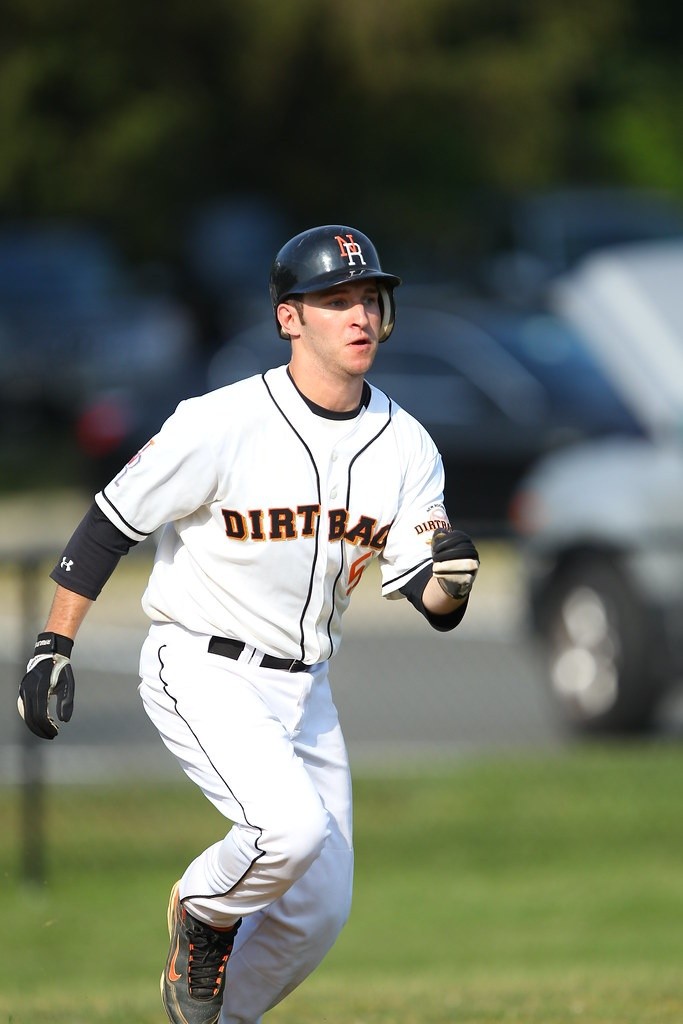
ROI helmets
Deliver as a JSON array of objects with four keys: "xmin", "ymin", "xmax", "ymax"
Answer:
[{"xmin": 268, "ymin": 226, "xmax": 402, "ymax": 341}]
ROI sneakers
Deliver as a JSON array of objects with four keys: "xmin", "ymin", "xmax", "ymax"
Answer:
[{"xmin": 158, "ymin": 880, "xmax": 243, "ymax": 1024}]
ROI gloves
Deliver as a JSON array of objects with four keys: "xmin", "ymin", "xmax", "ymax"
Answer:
[
  {"xmin": 16, "ymin": 633, "xmax": 76, "ymax": 739},
  {"xmin": 432, "ymin": 528, "xmax": 480, "ymax": 601}
]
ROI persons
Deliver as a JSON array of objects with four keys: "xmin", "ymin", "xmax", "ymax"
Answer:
[{"xmin": 14, "ymin": 225, "xmax": 479, "ymax": 1024}]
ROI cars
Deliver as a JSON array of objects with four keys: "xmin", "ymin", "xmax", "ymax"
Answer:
[{"xmin": 515, "ymin": 439, "xmax": 683, "ymax": 740}]
